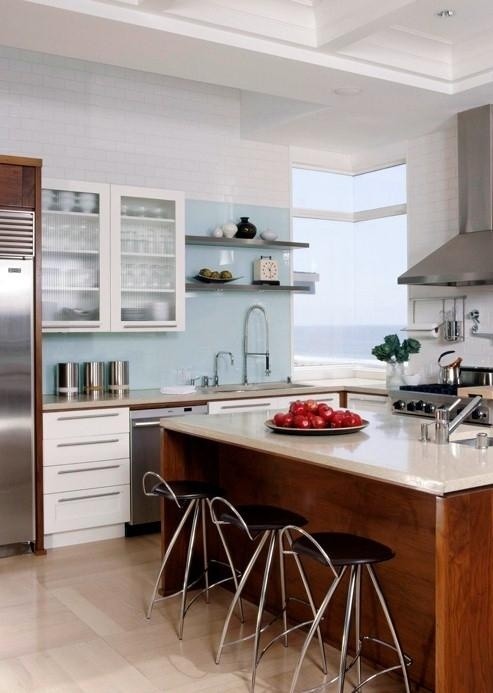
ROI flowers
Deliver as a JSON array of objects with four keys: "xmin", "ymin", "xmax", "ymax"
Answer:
[{"xmin": 372, "ymin": 334, "xmax": 423, "ymax": 364}]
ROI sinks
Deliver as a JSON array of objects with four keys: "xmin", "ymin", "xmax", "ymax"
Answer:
[
  {"xmin": 251, "ymin": 383, "xmax": 316, "ymax": 391},
  {"xmin": 451, "ymin": 434, "xmax": 493, "ymax": 448},
  {"xmin": 206, "ymin": 384, "xmax": 251, "ymax": 392}
]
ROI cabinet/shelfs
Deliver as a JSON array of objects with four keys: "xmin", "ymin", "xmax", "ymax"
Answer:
[
  {"xmin": 43, "ymin": 181, "xmax": 186, "ymax": 333},
  {"xmin": 45, "ymin": 394, "xmax": 133, "ymax": 552},
  {"xmin": 204, "ymin": 380, "xmax": 344, "ymax": 415},
  {"xmin": 186, "ymin": 235, "xmax": 310, "ymax": 293}
]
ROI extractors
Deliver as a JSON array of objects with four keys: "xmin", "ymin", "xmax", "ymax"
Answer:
[{"xmin": 397, "ymin": 105, "xmax": 493, "ymax": 286}]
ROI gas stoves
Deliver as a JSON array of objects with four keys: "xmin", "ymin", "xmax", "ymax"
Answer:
[{"xmin": 392, "ymin": 382, "xmax": 493, "ymax": 426}]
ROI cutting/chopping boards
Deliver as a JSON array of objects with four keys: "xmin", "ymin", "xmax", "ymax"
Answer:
[{"xmin": 457, "ymin": 384, "xmax": 492, "ymax": 400}]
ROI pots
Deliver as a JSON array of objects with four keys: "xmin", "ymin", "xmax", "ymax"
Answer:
[{"xmin": 461, "ymin": 368, "xmax": 492, "ymax": 385}]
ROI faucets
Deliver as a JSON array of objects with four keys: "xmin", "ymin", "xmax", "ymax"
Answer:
[
  {"xmin": 242, "ymin": 306, "xmax": 270, "ymax": 385},
  {"xmin": 434, "ymin": 396, "xmax": 482, "ymax": 444},
  {"xmin": 214, "ymin": 352, "xmax": 234, "ymax": 385}
]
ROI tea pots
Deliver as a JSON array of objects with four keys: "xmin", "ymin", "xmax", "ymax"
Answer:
[{"xmin": 437, "ymin": 350, "xmax": 462, "ymax": 384}]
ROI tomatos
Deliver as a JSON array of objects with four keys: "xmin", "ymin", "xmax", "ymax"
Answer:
[{"xmin": 271, "ymin": 399, "xmax": 362, "ymax": 429}]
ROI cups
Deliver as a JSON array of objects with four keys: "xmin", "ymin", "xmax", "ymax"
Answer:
[
  {"xmin": 137, "ymin": 233, "xmax": 147, "ymax": 253},
  {"xmin": 159, "ymin": 234, "xmax": 173, "ymax": 255},
  {"xmin": 146, "ymin": 228, "xmax": 154, "ymax": 254},
  {"xmin": 121, "ymin": 230, "xmax": 136, "ymax": 253}
]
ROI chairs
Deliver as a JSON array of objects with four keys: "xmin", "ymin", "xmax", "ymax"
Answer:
[
  {"xmin": 144, "ymin": 475, "xmax": 244, "ymax": 640},
  {"xmin": 279, "ymin": 523, "xmax": 418, "ymax": 692},
  {"xmin": 204, "ymin": 499, "xmax": 333, "ymax": 692}
]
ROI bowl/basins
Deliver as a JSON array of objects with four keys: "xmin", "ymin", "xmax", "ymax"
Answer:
[
  {"xmin": 153, "ymin": 302, "xmax": 169, "ymax": 319},
  {"xmin": 79, "ymin": 194, "xmax": 96, "ymax": 211},
  {"xmin": 41, "ymin": 190, "xmax": 53, "ymax": 209},
  {"xmin": 56, "ymin": 192, "xmax": 76, "ymax": 211}
]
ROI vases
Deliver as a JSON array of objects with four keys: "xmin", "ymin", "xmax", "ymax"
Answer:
[{"xmin": 389, "ymin": 362, "xmax": 427, "ymax": 400}]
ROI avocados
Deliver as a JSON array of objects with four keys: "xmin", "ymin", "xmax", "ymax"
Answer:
[
  {"xmin": 199, "ymin": 268, "xmax": 211, "ymax": 278},
  {"xmin": 211, "ymin": 271, "xmax": 220, "ymax": 279},
  {"xmin": 221, "ymin": 270, "xmax": 232, "ymax": 279}
]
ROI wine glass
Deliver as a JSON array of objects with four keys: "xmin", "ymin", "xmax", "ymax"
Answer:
[
  {"xmin": 162, "ymin": 265, "xmax": 175, "ymax": 289},
  {"xmin": 122, "ymin": 263, "xmax": 135, "ymax": 288},
  {"xmin": 137, "ymin": 263, "xmax": 150, "ymax": 288},
  {"xmin": 152, "ymin": 265, "xmax": 162, "ymax": 287}
]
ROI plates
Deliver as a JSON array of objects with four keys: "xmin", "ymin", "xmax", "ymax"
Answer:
[
  {"xmin": 266, "ymin": 420, "xmax": 370, "ymax": 432},
  {"xmin": 122, "ymin": 307, "xmax": 145, "ymax": 320},
  {"xmin": 69, "ymin": 269, "xmax": 94, "ymax": 286},
  {"xmin": 192, "ymin": 273, "xmax": 245, "ymax": 283}
]
